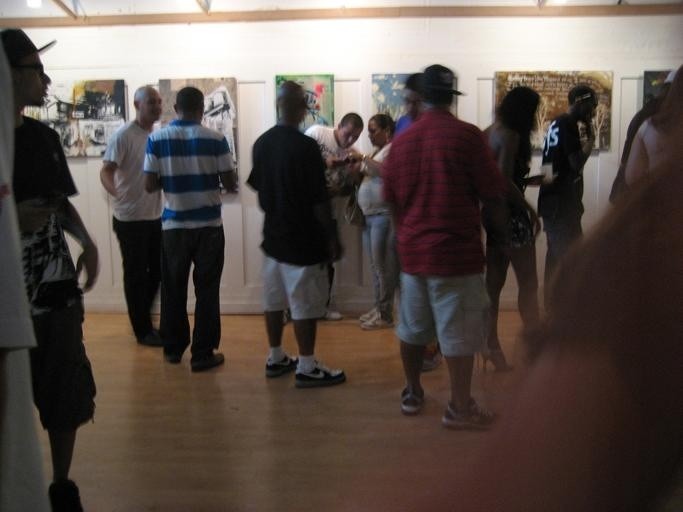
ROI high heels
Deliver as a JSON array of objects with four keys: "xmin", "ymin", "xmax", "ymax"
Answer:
[{"xmin": 482, "ymin": 338, "xmax": 513, "ymax": 372}]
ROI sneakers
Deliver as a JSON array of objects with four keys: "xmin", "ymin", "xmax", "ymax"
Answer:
[
  {"xmin": 49, "ymin": 480, "xmax": 83, "ymax": 512},
  {"xmin": 422, "ymin": 351, "xmax": 442, "ymax": 371},
  {"xmin": 360, "ymin": 307, "xmax": 393, "ymax": 330},
  {"xmin": 139, "ymin": 329, "xmax": 163, "ymax": 345},
  {"xmin": 401, "ymin": 386, "xmax": 424, "ymax": 415},
  {"xmin": 192, "ymin": 354, "xmax": 224, "ymax": 370},
  {"xmin": 166, "ymin": 340, "xmax": 190, "ymax": 363},
  {"xmin": 295, "ymin": 360, "xmax": 345, "ymax": 387},
  {"xmin": 442, "ymin": 396, "xmax": 495, "ymax": 428},
  {"xmin": 266, "ymin": 352, "xmax": 298, "ymax": 377},
  {"xmin": 324, "ymin": 309, "xmax": 343, "ymax": 321}
]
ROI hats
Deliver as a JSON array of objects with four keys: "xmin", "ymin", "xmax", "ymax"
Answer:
[
  {"xmin": 422, "ymin": 65, "xmax": 461, "ymax": 95},
  {"xmin": 0, "ymin": 29, "xmax": 56, "ymax": 64}
]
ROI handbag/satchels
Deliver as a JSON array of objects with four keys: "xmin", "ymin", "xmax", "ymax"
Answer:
[
  {"xmin": 504, "ymin": 208, "xmax": 535, "ymax": 258},
  {"xmin": 344, "ymin": 193, "xmax": 365, "ymax": 226}
]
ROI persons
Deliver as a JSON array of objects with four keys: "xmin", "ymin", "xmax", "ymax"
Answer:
[
  {"xmin": 0, "ymin": 27, "xmax": 99, "ymax": 511},
  {"xmin": 0, "ymin": 47, "xmax": 53, "ymax": 511},
  {"xmin": 282, "ymin": 66, "xmax": 682, "ymax": 379},
  {"xmin": 384, "ymin": 66, "xmax": 541, "ymax": 427},
  {"xmin": 244, "ymin": 80, "xmax": 347, "ymax": 389},
  {"xmin": 142, "ymin": 87, "xmax": 237, "ymax": 374},
  {"xmin": 97, "ymin": 85, "xmax": 165, "ymax": 348}
]
